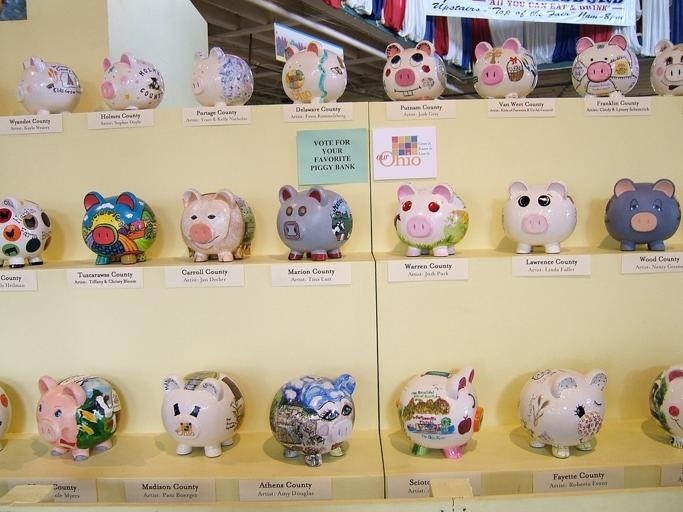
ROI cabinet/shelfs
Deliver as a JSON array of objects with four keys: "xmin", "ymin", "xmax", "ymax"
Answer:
[{"xmin": 0, "ymin": 96, "xmax": 682, "ymax": 512}]
